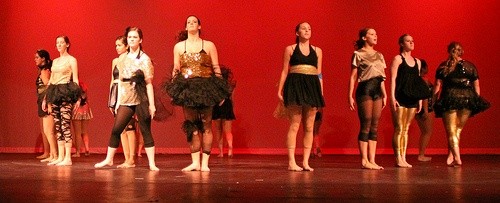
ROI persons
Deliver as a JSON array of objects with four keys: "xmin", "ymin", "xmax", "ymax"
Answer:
[
  {"xmin": 42, "ymin": 35, "xmax": 78, "ymax": 166},
  {"xmin": 33, "ymin": 50, "xmax": 58, "ymax": 162},
  {"xmin": 349, "ymin": 27, "xmax": 387, "ymax": 169},
  {"xmin": 71, "ymin": 72, "xmax": 93, "ymax": 157},
  {"xmin": 391, "ymin": 33, "xmax": 434, "ymax": 168},
  {"xmin": 211, "ymin": 67, "xmax": 236, "ymax": 158},
  {"xmin": 272, "ymin": 22, "xmax": 323, "ymax": 172},
  {"xmin": 94, "ymin": 26, "xmax": 160, "ymax": 171},
  {"xmin": 172, "ymin": 15, "xmax": 221, "ymax": 172},
  {"xmin": 433, "ymin": 42, "xmax": 491, "ymax": 167}
]
